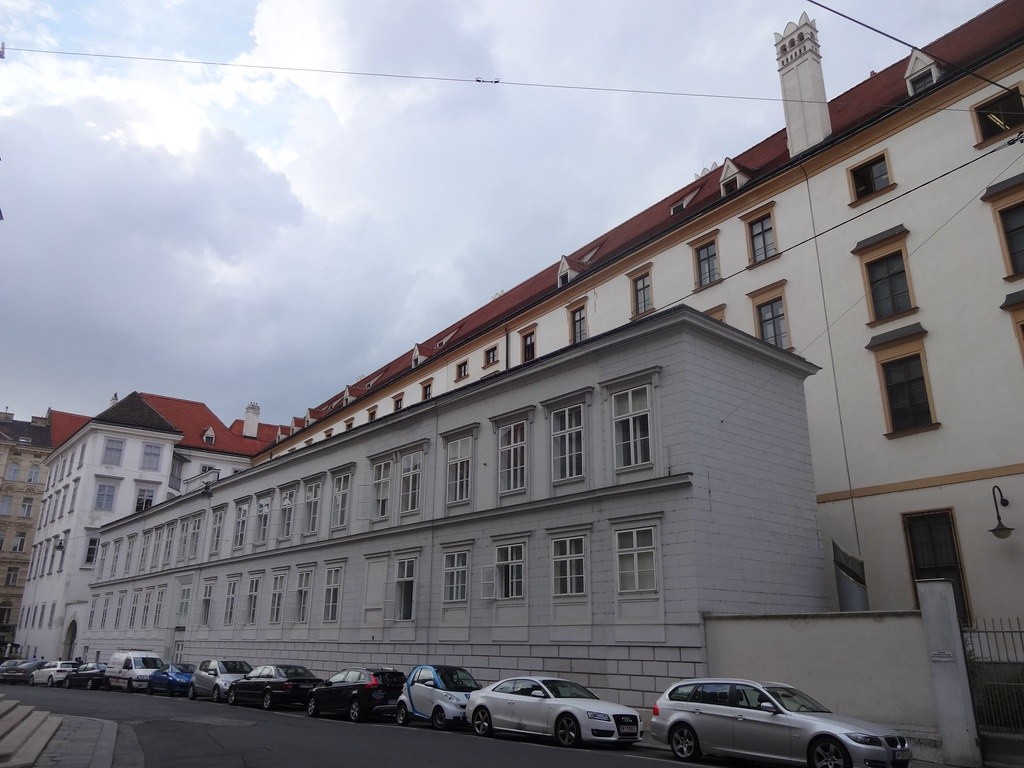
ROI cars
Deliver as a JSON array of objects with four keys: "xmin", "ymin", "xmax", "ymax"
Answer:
[
  {"xmin": 147, "ymin": 663, "xmax": 199, "ymax": 697},
  {"xmin": 29, "ymin": 660, "xmax": 81, "ymax": 687},
  {"xmin": 465, "ymin": 675, "xmax": 644, "ymax": 748},
  {"xmin": 62, "ymin": 662, "xmax": 107, "ymax": 690},
  {"xmin": 226, "ymin": 664, "xmax": 324, "ymax": 711},
  {"xmin": 0, "ymin": 659, "xmax": 29, "ymax": 681},
  {"xmin": 395, "ymin": 664, "xmax": 482, "ymax": 731},
  {"xmin": 188, "ymin": 658, "xmax": 254, "ymax": 703},
  {"xmin": 3, "ymin": 661, "xmax": 48, "ymax": 685}
]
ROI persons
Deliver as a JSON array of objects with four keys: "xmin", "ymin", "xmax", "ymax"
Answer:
[
  {"xmin": 294, "ymin": 668, "xmax": 300, "ymax": 676},
  {"xmin": 444, "ymin": 672, "xmax": 454, "ymax": 688},
  {"xmin": 232, "ymin": 663, "xmax": 238, "ymax": 671},
  {"xmin": 76, "ymin": 656, "xmax": 83, "ymax": 665},
  {"xmin": 41, "ymin": 656, "xmax": 44, "ymax": 660},
  {"xmin": 394, "ymin": 673, "xmax": 404, "ymax": 684},
  {"xmin": 546, "ymin": 683, "xmax": 558, "ymax": 698},
  {"xmin": 769, "ymin": 692, "xmax": 785, "ymax": 711},
  {"xmin": 59, "ymin": 656, "xmax": 62, "ymax": 660},
  {"xmin": 151, "ymin": 660, "xmax": 156, "ymax": 668},
  {"xmin": 32, "ymin": 655, "xmax": 37, "ymax": 661}
]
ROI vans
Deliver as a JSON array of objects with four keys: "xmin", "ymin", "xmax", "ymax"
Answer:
[{"xmin": 102, "ymin": 649, "xmax": 164, "ymax": 692}]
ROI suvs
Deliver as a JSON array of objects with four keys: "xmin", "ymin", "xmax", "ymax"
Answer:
[
  {"xmin": 649, "ymin": 678, "xmax": 911, "ymax": 768},
  {"xmin": 304, "ymin": 667, "xmax": 408, "ymax": 722}
]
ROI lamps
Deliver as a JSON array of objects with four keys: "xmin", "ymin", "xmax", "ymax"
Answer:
[
  {"xmin": 201, "ymin": 483, "xmax": 213, "ymax": 497},
  {"xmin": 55, "ymin": 540, "xmax": 64, "ymax": 551},
  {"xmin": 987, "ymin": 485, "xmax": 1016, "ymax": 540}
]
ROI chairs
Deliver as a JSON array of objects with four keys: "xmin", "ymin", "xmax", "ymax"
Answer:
[
  {"xmin": 737, "ymin": 690, "xmax": 745, "ymax": 706},
  {"xmin": 230, "ymin": 663, "xmax": 239, "ymax": 671},
  {"xmin": 718, "ymin": 690, "xmax": 728, "ymax": 705},
  {"xmin": 756, "ymin": 693, "xmax": 769, "ymax": 710}
]
